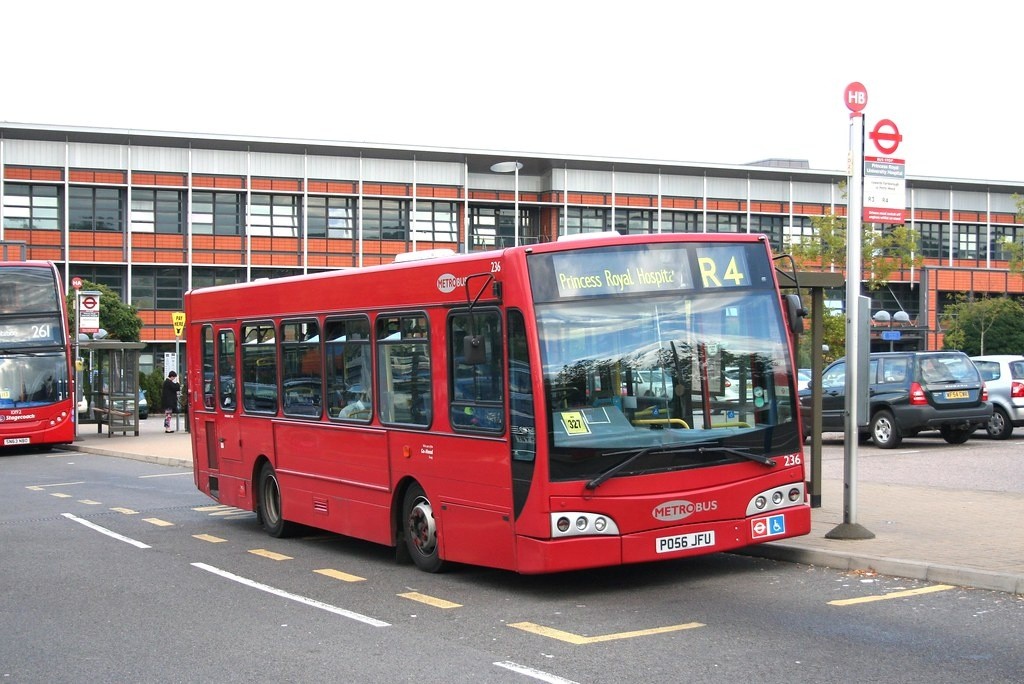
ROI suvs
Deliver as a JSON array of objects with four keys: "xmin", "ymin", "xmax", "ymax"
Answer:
[{"xmin": 776, "ymin": 351, "xmax": 992, "ymax": 450}]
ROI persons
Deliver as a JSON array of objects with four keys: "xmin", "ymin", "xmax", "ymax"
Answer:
[
  {"xmin": 339, "ymin": 399, "xmax": 371, "ymax": 420},
  {"xmin": 41, "ymin": 375, "xmax": 56, "ymax": 402},
  {"xmin": 162, "ymin": 371, "xmax": 180, "ymax": 432}
]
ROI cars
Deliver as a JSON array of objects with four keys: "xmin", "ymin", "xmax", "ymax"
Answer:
[
  {"xmin": 939, "ymin": 354, "xmax": 1024, "ymax": 440},
  {"xmin": 203, "ymin": 352, "xmax": 535, "ymax": 462},
  {"xmin": 690, "ymin": 364, "xmax": 844, "ymax": 416},
  {"xmin": 77, "ymin": 377, "xmax": 148, "ymax": 419},
  {"xmin": 584, "ymin": 375, "xmax": 628, "ymax": 398},
  {"xmin": 620, "ymin": 369, "xmax": 674, "ymax": 401}
]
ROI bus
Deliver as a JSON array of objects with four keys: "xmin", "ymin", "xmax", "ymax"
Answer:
[
  {"xmin": 184, "ymin": 229, "xmax": 811, "ymax": 574},
  {"xmin": 0, "ymin": 258, "xmax": 84, "ymax": 452}
]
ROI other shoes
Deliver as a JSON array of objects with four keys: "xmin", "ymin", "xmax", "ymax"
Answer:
[{"xmin": 165, "ymin": 431, "xmax": 175, "ymax": 433}]
